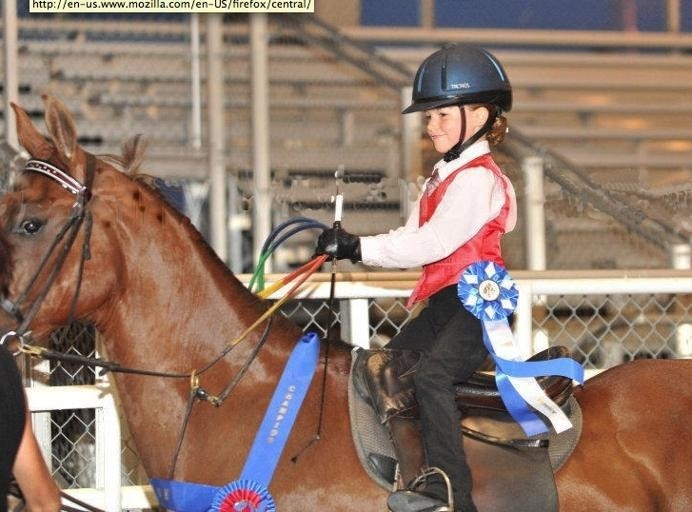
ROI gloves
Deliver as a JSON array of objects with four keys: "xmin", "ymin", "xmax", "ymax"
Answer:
[{"xmin": 315, "ymin": 227, "xmax": 361, "ymax": 265}]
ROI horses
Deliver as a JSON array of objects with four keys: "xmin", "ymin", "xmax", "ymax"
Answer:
[{"xmin": 0, "ymin": 93, "xmax": 692, "ymax": 512}]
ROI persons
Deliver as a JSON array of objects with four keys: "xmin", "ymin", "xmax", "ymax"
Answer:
[
  {"xmin": 313, "ymin": 37, "xmax": 520, "ymax": 511},
  {"xmin": 0, "ymin": 340, "xmax": 65, "ymax": 512}
]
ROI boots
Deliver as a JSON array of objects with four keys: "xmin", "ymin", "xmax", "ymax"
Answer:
[{"xmin": 387, "ymin": 463, "xmax": 478, "ymax": 512}]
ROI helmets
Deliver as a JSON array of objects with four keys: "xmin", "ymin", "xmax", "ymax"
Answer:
[{"xmin": 400, "ymin": 41, "xmax": 513, "ymax": 115}]
